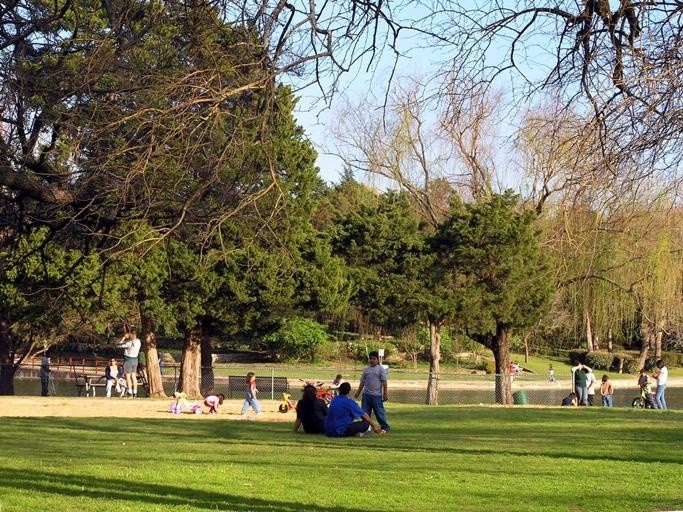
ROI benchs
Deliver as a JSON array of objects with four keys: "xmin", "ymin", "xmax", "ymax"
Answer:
[
  {"xmin": 75, "ymin": 372, "xmax": 150, "ymax": 398},
  {"xmin": 227, "ymin": 374, "xmax": 289, "ymax": 400}
]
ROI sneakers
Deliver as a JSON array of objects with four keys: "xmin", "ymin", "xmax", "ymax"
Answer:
[
  {"xmin": 132, "ymin": 392, "xmax": 138, "ymax": 398},
  {"xmin": 122, "ymin": 391, "xmax": 133, "ymax": 398}
]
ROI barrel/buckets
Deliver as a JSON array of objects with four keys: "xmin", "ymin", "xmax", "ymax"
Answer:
[{"xmin": 513, "ymin": 391, "xmax": 529, "ymax": 405}]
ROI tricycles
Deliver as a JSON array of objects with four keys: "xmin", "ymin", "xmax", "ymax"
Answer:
[{"xmin": 278, "ymin": 392, "xmax": 297, "ymax": 413}]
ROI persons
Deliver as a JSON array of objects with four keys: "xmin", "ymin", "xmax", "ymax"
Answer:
[
  {"xmin": 241, "ymin": 372, "xmax": 263, "ymax": 414},
  {"xmin": 204, "ymin": 393, "xmax": 224, "ymax": 414},
  {"xmin": 561, "ymin": 359, "xmax": 613, "ymax": 407},
  {"xmin": 101, "ymin": 328, "xmax": 141, "ymax": 397},
  {"xmin": 638, "ymin": 360, "xmax": 668, "ymax": 409},
  {"xmin": 547, "ymin": 364, "xmax": 556, "ymax": 383},
  {"xmin": 158, "ymin": 351, "xmax": 164, "ymax": 375},
  {"xmin": 510, "ymin": 361, "xmax": 522, "ymax": 375},
  {"xmin": 40, "ymin": 355, "xmax": 51, "ymax": 396},
  {"xmin": 292, "ymin": 351, "xmax": 391, "ymax": 437}
]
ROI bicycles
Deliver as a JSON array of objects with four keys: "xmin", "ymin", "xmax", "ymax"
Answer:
[{"xmin": 631, "ymin": 382, "xmax": 657, "ymax": 408}]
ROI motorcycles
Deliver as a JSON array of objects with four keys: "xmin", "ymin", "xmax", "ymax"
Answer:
[{"xmin": 296, "ymin": 378, "xmax": 341, "ymax": 408}]
